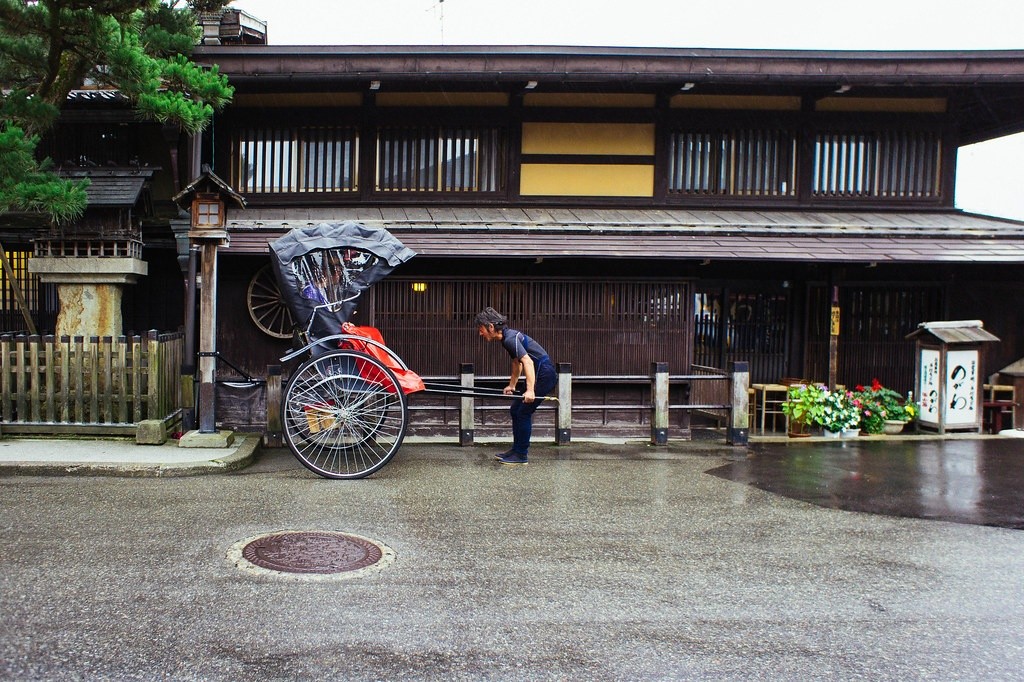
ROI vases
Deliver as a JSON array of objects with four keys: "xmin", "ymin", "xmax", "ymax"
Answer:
[
  {"xmin": 842, "ymin": 427, "xmax": 864, "ymax": 437},
  {"xmin": 823, "ymin": 428, "xmax": 840, "ymax": 438},
  {"xmin": 883, "ymin": 418, "xmax": 908, "ymax": 434}
]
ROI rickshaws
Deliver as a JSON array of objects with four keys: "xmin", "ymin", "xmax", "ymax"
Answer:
[{"xmin": 267, "ymin": 219, "xmax": 561, "ymax": 480}]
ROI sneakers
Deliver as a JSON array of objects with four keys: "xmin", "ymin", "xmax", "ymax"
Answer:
[
  {"xmin": 495, "ymin": 449, "xmax": 514, "ymax": 460},
  {"xmin": 499, "ymin": 452, "xmax": 528, "ymax": 465}
]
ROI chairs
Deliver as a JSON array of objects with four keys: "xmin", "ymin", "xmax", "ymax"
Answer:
[{"xmin": 343, "ymin": 325, "xmax": 424, "ymax": 394}]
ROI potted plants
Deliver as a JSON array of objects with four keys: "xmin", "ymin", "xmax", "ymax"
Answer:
[{"xmin": 781, "ymin": 384, "xmax": 819, "ymax": 436}]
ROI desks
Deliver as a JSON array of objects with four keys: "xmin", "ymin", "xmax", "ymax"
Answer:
[{"xmin": 753, "ymin": 383, "xmax": 789, "ymax": 434}]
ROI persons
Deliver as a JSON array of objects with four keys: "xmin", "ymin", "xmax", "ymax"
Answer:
[
  {"xmin": 302, "ymin": 262, "xmax": 339, "ymax": 309},
  {"xmin": 476, "ymin": 308, "xmax": 557, "ymax": 465}
]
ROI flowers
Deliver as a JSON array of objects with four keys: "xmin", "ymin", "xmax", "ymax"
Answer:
[{"xmin": 820, "ymin": 379, "xmax": 913, "ymax": 432}]
